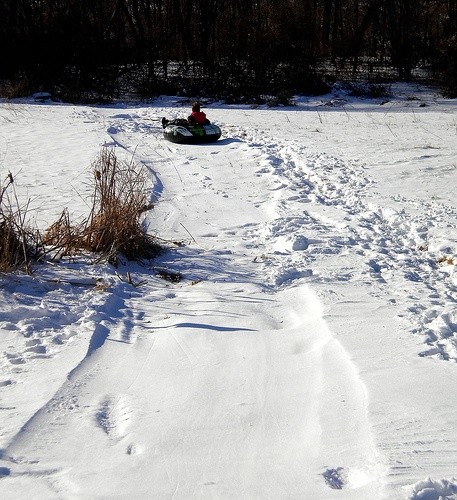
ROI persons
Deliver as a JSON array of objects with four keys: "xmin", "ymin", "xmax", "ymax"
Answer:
[{"xmin": 188, "ymin": 102, "xmax": 211, "ymax": 127}]
